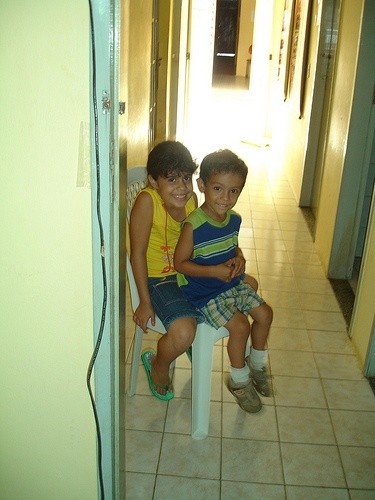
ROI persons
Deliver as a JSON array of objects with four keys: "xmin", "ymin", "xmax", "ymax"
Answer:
[
  {"xmin": 174, "ymin": 148, "xmax": 273, "ymax": 413},
  {"xmin": 132, "ymin": 141, "xmax": 259, "ymax": 400}
]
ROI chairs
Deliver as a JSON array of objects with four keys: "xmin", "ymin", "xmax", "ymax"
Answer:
[{"xmin": 123, "ymin": 166, "xmax": 248, "ymax": 440}]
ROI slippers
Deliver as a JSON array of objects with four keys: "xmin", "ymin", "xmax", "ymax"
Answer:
[
  {"xmin": 142, "ymin": 351, "xmax": 177, "ymax": 401},
  {"xmin": 186, "ymin": 346, "xmax": 193, "ymax": 361}
]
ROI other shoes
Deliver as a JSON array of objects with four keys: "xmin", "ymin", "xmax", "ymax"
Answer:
[
  {"xmin": 224, "ymin": 372, "xmax": 263, "ymax": 413},
  {"xmin": 245, "ymin": 356, "xmax": 271, "ymax": 398}
]
